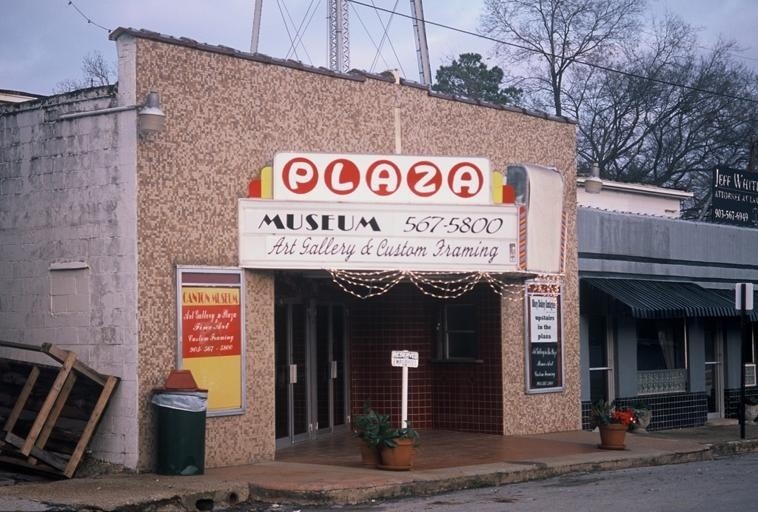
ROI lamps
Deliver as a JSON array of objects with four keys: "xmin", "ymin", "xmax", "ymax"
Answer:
[
  {"xmin": 135, "ymin": 85, "xmax": 167, "ymax": 136},
  {"xmin": 565, "ymin": 144, "xmax": 603, "ymax": 193}
]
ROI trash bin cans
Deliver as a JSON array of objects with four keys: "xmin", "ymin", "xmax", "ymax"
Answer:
[{"xmin": 151, "ymin": 370, "xmax": 208, "ymax": 476}]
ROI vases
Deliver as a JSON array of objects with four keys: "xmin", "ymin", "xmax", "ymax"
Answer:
[{"xmin": 598, "ymin": 424, "xmax": 628, "ymax": 449}]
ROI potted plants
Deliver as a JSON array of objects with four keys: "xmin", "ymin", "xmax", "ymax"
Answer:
[
  {"xmin": 631, "ymin": 402, "xmax": 653, "ymax": 432},
  {"xmin": 354, "ymin": 407, "xmax": 422, "ymax": 470},
  {"xmin": 744, "ymin": 396, "xmax": 758, "ymax": 425}
]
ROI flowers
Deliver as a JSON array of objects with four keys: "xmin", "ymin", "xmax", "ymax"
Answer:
[{"xmin": 587, "ymin": 399, "xmax": 642, "ymax": 428}]
